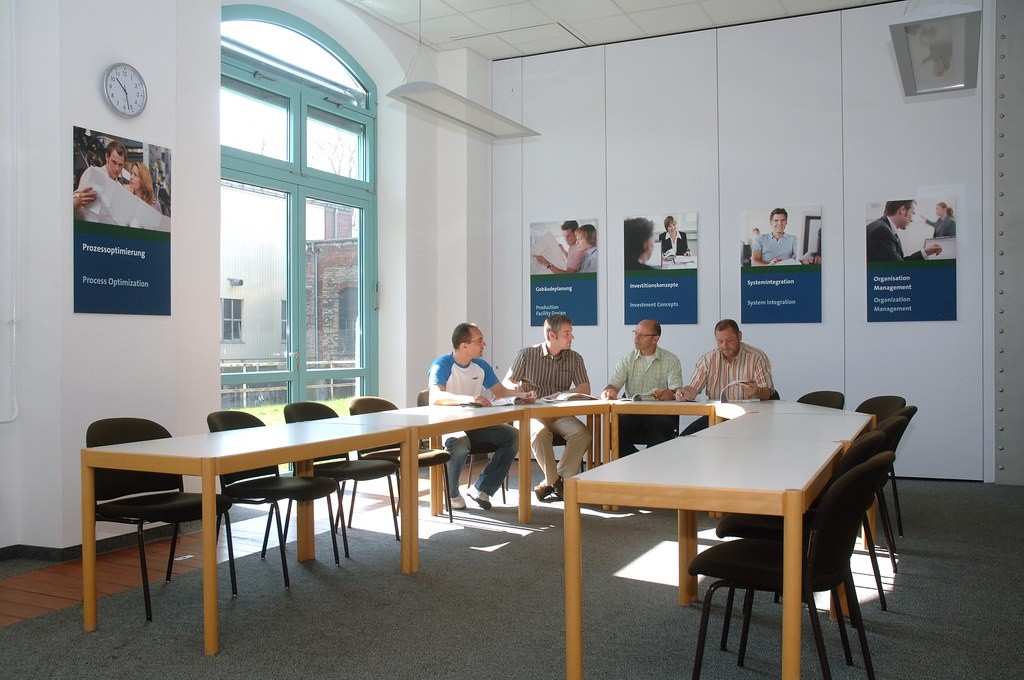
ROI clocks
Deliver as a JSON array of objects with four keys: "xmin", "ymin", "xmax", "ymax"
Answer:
[{"xmin": 103, "ymin": 62, "xmax": 147, "ymax": 117}]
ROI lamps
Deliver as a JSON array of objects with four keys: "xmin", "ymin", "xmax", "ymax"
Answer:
[
  {"xmin": 889, "ymin": 0, "xmax": 982, "ymax": 97},
  {"xmin": 385, "ymin": 0, "xmax": 541, "ymax": 138}
]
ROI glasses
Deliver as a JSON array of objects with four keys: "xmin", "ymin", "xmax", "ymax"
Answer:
[
  {"xmin": 459, "ymin": 339, "xmax": 485, "ymax": 344},
  {"xmin": 633, "ymin": 330, "xmax": 657, "ymax": 338}
]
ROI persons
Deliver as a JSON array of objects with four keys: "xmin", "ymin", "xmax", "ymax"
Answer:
[
  {"xmin": 73, "ymin": 140, "xmax": 126, "ymax": 220},
  {"xmin": 866, "ymin": 200, "xmax": 943, "ymax": 262},
  {"xmin": 128, "ymin": 162, "xmax": 162, "ymax": 214},
  {"xmin": 601, "ymin": 320, "xmax": 683, "ymax": 459},
  {"xmin": 501, "ymin": 314, "xmax": 591, "ymax": 502},
  {"xmin": 427, "ymin": 323, "xmax": 538, "ymax": 511},
  {"xmin": 751, "ymin": 208, "xmax": 798, "ymax": 266},
  {"xmin": 624, "ymin": 217, "xmax": 655, "ymax": 270},
  {"xmin": 659, "ymin": 215, "xmax": 692, "ymax": 257},
  {"xmin": 921, "ymin": 202, "xmax": 956, "ymax": 237},
  {"xmin": 532, "ymin": 221, "xmax": 584, "ymax": 274},
  {"xmin": 574, "ymin": 225, "xmax": 597, "ymax": 273},
  {"xmin": 675, "ymin": 319, "xmax": 775, "ymax": 436}
]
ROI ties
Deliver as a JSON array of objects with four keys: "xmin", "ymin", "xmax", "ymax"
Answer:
[{"xmin": 895, "ymin": 234, "xmax": 900, "ymax": 252}]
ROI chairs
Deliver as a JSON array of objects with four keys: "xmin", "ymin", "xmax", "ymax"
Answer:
[
  {"xmin": 416, "ymin": 389, "xmax": 505, "ymax": 507},
  {"xmin": 682, "ymin": 389, "xmax": 918, "ymax": 680},
  {"xmin": 207, "ymin": 411, "xmax": 338, "ymax": 589},
  {"xmin": 492, "ymin": 390, "xmax": 584, "ymax": 489},
  {"xmin": 347, "ymin": 395, "xmax": 451, "ymax": 531},
  {"xmin": 284, "ymin": 401, "xmax": 399, "ymax": 557},
  {"xmin": 86, "ymin": 418, "xmax": 238, "ymax": 623}
]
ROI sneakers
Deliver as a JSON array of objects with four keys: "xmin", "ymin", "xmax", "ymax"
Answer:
[
  {"xmin": 450, "ymin": 493, "xmax": 466, "ymax": 509},
  {"xmin": 467, "ymin": 485, "xmax": 491, "ymax": 511},
  {"xmin": 534, "ymin": 483, "xmax": 552, "ymax": 500},
  {"xmin": 554, "ymin": 476, "xmax": 563, "ymax": 500}
]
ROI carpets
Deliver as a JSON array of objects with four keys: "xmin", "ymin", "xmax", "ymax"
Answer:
[{"xmin": 0, "ymin": 464, "xmax": 1024, "ymax": 680}]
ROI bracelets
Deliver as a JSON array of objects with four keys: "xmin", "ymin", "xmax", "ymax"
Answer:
[{"xmin": 546, "ymin": 264, "xmax": 551, "ymax": 268}]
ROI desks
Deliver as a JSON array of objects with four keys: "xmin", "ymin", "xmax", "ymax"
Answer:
[
  {"xmin": 307, "ymin": 404, "xmax": 528, "ymax": 572},
  {"xmin": 564, "ymin": 437, "xmax": 844, "ymax": 680},
  {"xmin": 686, "ymin": 415, "xmax": 874, "ymax": 615},
  {"xmin": 596, "ymin": 398, "xmax": 757, "ymax": 518},
  {"xmin": 715, "ymin": 401, "xmax": 878, "ymax": 519},
  {"xmin": 80, "ymin": 423, "xmax": 410, "ymax": 656},
  {"xmin": 437, "ymin": 402, "xmax": 610, "ymax": 523}
]
